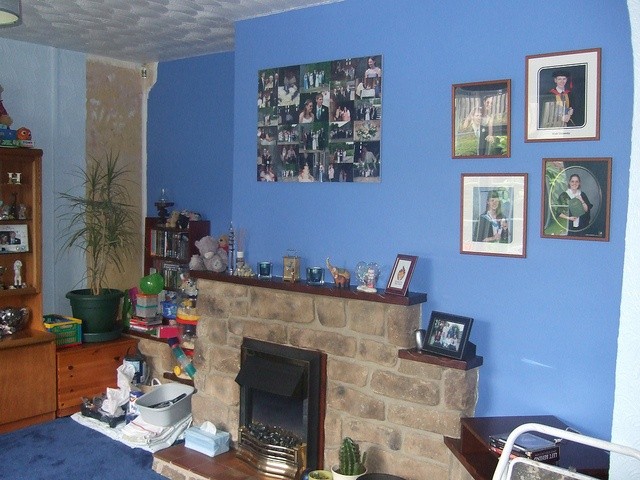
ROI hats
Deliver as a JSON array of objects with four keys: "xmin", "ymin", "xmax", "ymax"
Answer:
[
  {"xmin": 480, "ymin": 189, "xmax": 505, "ymax": 199},
  {"xmin": 552, "ymin": 70, "xmax": 572, "ymax": 88}
]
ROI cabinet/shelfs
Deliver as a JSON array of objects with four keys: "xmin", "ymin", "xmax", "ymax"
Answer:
[
  {"xmin": 57, "ymin": 339, "xmax": 141, "ymax": 417},
  {"xmin": 0, "ymin": 149, "xmax": 56, "ymax": 433},
  {"xmin": 145, "ymin": 217, "xmax": 211, "ymax": 294}
]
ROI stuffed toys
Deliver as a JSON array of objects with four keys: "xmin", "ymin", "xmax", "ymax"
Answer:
[
  {"xmin": 164, "ymin": 210, "xmax": 180, "ymax": 228},
  {"xmin": 177, "ymin": 209, "xmax": 190, "ymax": 229},
  {"xmin": 219, "ymin": 234, "xmax": 229, "ymax": 252},
  {"xmin": 187, "ymin": 210, "xmax": 202, "ymax": 229},
  {"xmin": 189, "ymin": 236, "xmax": 229, "ymax": 272},
  {"xmin": 326, "ymin": 257, "xmax": 351, "ymax": 288},
  {"xmin": 9, "ymin": 260, "xmax": 27, "ymax": 290},
  {"xmin": 332, "ymin": 272, "xmax": 347, "ymax": 288}
]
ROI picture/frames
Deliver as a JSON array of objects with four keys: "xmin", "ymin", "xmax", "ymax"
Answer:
[
  {"xmin": 540, "ymin": 158, "xmax": 612, "ymax": 242},
  {"xmin": 452, "ymin": 79, "xmax": 511, "ymax": 158},
  {"xmin": 386, "ymin": 254, "xmax": 418, "ymax": 298},
  {"xmin": 460, "ymin": 174, "xmax": 527, "ymax": 258},
  {"xmin": 0, "ymin": 225, "xmax": 28, "ymax": 251},
  {"xmin": 525, "ymin": 47, "xmax": 601, "ymax": 140},
  {"xmin": 421, "ymin": 310, "xmax": 474, "ymax": 360}
]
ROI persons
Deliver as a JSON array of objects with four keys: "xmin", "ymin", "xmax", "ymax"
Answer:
[
  {"xmin": 556, "ymin": 173, "xmax": 594, "ymax": 231},
  {"xmin": 257, "ymin": 56, "xmax": 381, "ymax": 182},
  {"xmin": 0, "ymin": 230, "xmax": 8, "ymax": 245},
  {"xmin": 464, "ymin": 94, "xmax": 496, "ymax": 156},
  {"xmin": 429, "ymin": 318, "xmax": 462, "ymax": 352},
  {"xmin": 544, "ymin": 71, "xmax": 576, "ymax": 128},
  {"xmin": 8, "ymin": 231, "xmax": 21, "ymax": 245},
  {"xmin": 473, "ymin": 190, "xmax": 509, "ymax": 244}
]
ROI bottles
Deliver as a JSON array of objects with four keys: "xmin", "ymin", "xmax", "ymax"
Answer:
[{"xmin": 236, "ymin": 252, "xmax": 245, "ymax": 269}]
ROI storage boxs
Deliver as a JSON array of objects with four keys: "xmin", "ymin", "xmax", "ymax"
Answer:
[
  {"xmin": 136, "ymin": 306, "xmax": 158, "ymax": 318},
  {"xmin": 43, "ymin": 314, "xmax": 83, "ymax": 349},
  {"xmin": 136, "ymin": 294, "xmax": 157, "ymax": 306},
  {"xmin": 133, "ymin": 382, "xmax": 195, "ymax": 428}
]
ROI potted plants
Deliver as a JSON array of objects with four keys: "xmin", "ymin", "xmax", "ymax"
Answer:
[
  {"xmin": 51, "ymin": 149, "xmax": 144, "ymax": 343},
  {"xmin": 331, "ymin": 437, "xmax": 370, "ymax": 478}
]
